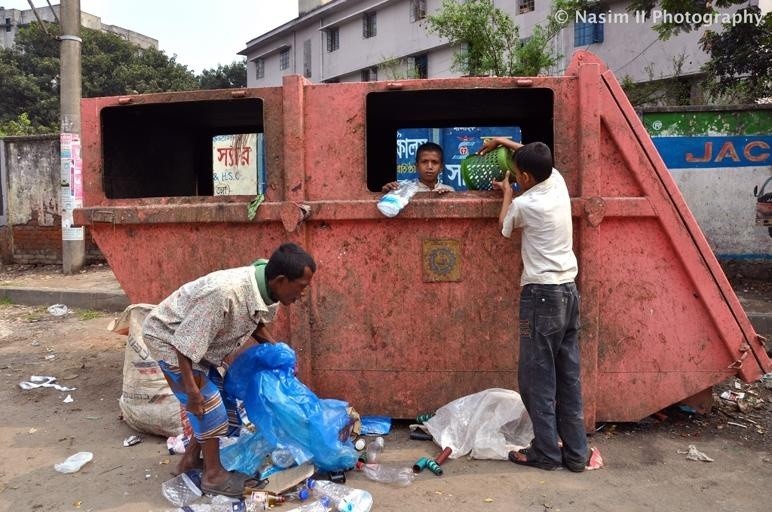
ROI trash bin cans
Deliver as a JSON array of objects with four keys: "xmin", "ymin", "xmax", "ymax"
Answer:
[{"xmin": 460, "ymin": 146, "xmax": 517, "ymax": 191}]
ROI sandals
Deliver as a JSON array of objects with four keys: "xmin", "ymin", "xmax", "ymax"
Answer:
[{"xmin": 508, "ymin": 447, "xmax": 558, "ymax": 471}]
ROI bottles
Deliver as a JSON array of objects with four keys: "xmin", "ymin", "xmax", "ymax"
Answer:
[{"xmin": 161, "ymin": 438, "xmax": 415, "ymax": 512}]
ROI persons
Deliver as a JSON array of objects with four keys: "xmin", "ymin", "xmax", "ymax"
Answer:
[
  {"xmin": 381, "ymin": 141, "xmax": 456, "ymax": 196},
  {"xmin": 477, "ymin": 136, "xmax": 588, "ymax": 473},
  {"xmin": 142, "ymin": 239, "xmax": 319, "ymax": 497}
]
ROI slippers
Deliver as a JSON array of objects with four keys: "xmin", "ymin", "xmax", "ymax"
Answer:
[{"xmin": 200, "ymin": 469, "xmax": 264, "ymax": 496}]
